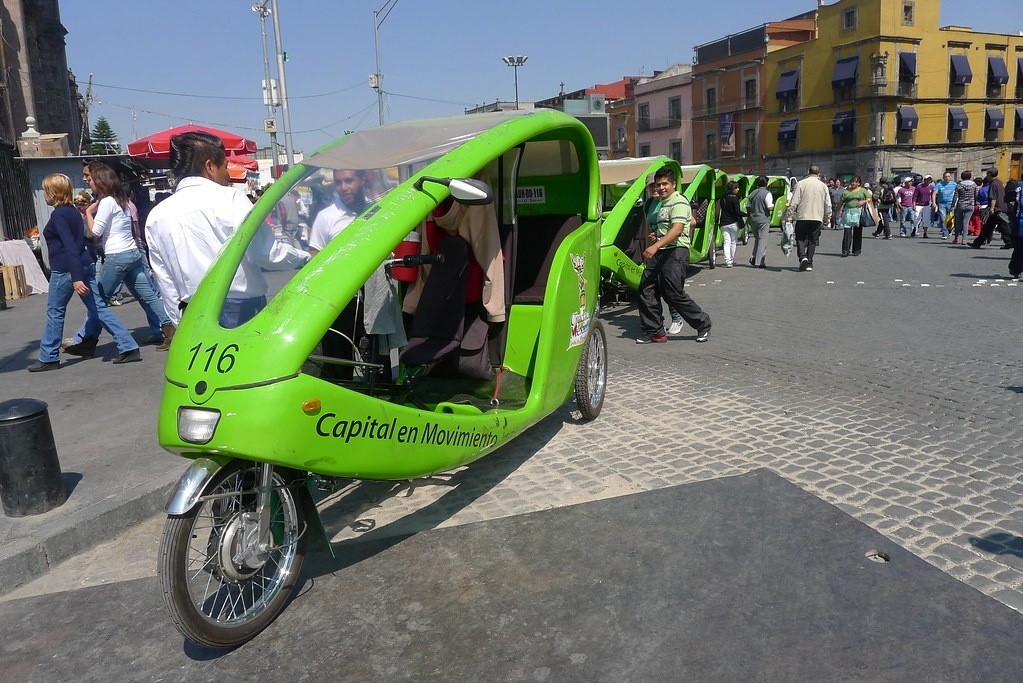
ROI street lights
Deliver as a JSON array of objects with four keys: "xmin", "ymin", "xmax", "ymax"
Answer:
[{"xmin": 502, "ymin": 56, "xmax": 529, "ymax": 110}]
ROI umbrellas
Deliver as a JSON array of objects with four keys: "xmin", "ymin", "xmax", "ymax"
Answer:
[
  {"xmin": 127, "ymin": 120, "xmax": 257, "ymax": 157},
  {"xmin": 226, "ymin": 151, "xmax": 259, "ymax": 172},
  {"xmin": 226, "ymin": 166, "xmax": 255, "ymax": 184}
]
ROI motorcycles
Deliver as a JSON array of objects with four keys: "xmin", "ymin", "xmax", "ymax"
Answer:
[
  {"xmin": 594, "ymin": 154, "xmax": 795, "ymax": 320},
  {"xmin": 154, "ymin": 107, "xmax": 609, "ymax": 652}
]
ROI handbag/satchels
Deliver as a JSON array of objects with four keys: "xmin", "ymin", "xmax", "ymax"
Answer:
[
  {"xmin": 780, "ymin": 221, "xmax": 795, "ymax": 258},
  {"xmin": 860, "ymin": 200, "xmax": 881, "ymax": 227},
  {"xmin": 736, "ymin": 217, "xmax": 745, "ymax": 229},
  {"xmin": 944, "ymin": 212, "xmax": 954, "ymax": 230}
]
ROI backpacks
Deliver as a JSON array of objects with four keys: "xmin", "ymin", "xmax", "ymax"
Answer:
[{"xmin": 879, "ymin": 185, "xmax": 896, "ymax": 205}]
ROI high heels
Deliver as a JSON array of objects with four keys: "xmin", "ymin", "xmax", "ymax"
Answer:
[
  {"xmin": 27, "ymin": 361, "xmax": 59, "ymax": 372},
  {"xmin": 113, "ymin": 348, "xmax": 140, "ymax": 363}
]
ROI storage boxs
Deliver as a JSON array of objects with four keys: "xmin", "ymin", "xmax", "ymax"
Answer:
[{"xmin": 17, "ymin": 133, "xmax": 71, "ymax": 156}]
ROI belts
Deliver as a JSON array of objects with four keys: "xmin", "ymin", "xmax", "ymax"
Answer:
[{"xmin": 223, "ymin": 303, "xmax": 262, "ymax": 313}]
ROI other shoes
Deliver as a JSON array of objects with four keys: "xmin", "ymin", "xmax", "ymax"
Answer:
[
  {"xmin": 852, "ymin": 251, "xmax": 861, "ymax": 255},
  {"xmin": 726, "ymin": 264, "xmax": 733, "ymax": 267},
  {"xmin": 61, "ymin": 338, "xmax": 74, "ymax": 349},
  {"xmin": 842, "ymin": 253, "xmax": 849, "ymax": 256},
  {"xmin": 141, "ymin": 336, "xmax": 164, "ymax": 345},
  {"xmin": 798, "ymin": 258, "xmax": 809, "ymax": 272},
  {"xmin": 806, "ymin": 266, "xmax": 812, "ymax": 271},
  {"xmin": 749, "ymin": 258, "xmax": 755, "ymax": 265},
  {"xmin": 756, "ymin": 264, "xmax": 767, "ymax": 268},
  {"xmin": 872, "ymin": 230, "xmax": 1022, "ymax": 278}
]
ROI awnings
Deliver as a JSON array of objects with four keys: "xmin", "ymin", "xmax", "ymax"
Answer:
[
  {"xmin": 985, "ymin": 107, "xmax": 1004, "ymax": 129},
  {"xmin": 830, "ymin": 57, "xmax": 859, "ymax": 88},
  {"xmin": 899, "ymin": 51, "xmax": 920, "ymax": 81},
  {"xmin": 832, "ymin": 109, "xmax": 856, "ymax": 136},
  {"xmin": 897, "ymin": 104, "xmax": 918, "ymax": 130},
  {"xmin": 949, "ymin": 55, "xmax": 973, "ymax": 84},
  {"xmin": 988, "ymin": 56, "xmax": 1009, "ymax": 84},
  {"xmin": 775, "ymin": 68, "xmax": 799, "ymax": 101},
  {"xmin": 777, "ymin": 118, "xmax": 799, "ymax": 142},
  {"xmin": 947, "ymin": 107, "xmax": 968, "ymax": 130},
  {"xmin": 1015, "ymin": 57, "xmax": 1023, "ymax": 130}
]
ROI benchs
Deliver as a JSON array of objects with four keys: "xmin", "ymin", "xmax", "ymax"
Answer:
[
  {"xmin": 685, "ymin": 195, "xmax": 709, "ymax": 226},
  {"xmin": 421, "ymin": 207, "xmax": 577, "ymax": 303}
]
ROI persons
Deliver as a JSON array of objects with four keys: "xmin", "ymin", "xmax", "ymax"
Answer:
[
  {"xmin": 719, "ymin": 180, "xmax": 745, "ymax": 268},
  {"xmin": 26, "ymin": 161, "xmax": 175, "ymax": 373},
  {"xmin": 783, "ymin": 166, "xmax": 1023, "ymax": 281},
  {"xmin": 748, "ymin": 175, "xmax": 775, "ymax": 269},
  {"xmin": 245, "ymin": 168, "xmax": 408, "ymax": 387},
  {"xmin": 145, "ymin": 130, "xmax": 319, "ymax": 330},
  {"xmin": 635, "ymin": 169, "xmax": 712, "ymax": 343}
]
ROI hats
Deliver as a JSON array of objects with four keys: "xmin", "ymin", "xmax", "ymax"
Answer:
[
  {"xmin": 646, "ymin": 172, "xmax": 655, "ymax": 186},
  {"xmin": 924, "ymin": 175, "xmax": 932, "ymax": 180},
  {"xmin": 863, "ymin": 182, "xmax": 870, "ymax": 187},
  {"xmin": 905, "ymin": 177, "xmax": 914, "ymax": 182}
]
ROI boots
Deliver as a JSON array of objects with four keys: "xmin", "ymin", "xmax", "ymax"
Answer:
[
  {"xmin": 156, "ymin": 325, "xmax": 176, "ymax": 351},
  {"xmin": 65, "ymin": 337, "xmax": 99, "ymax": 359}
]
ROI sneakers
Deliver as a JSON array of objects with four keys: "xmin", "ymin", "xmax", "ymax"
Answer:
[
  {"xmin": 696, "ymin": 330, "xmax": 711, "ymax": 341},
  {"xmin": 668, "ymin": 319, "xmax": 683, "ymax": 334},
  {"xmin": 636, "ymin": 333, "xmax": 667, "ymax": 343},
  {"xmin": 664, "ymin": 326, "xmax": 667, "ymax": 332}
]
